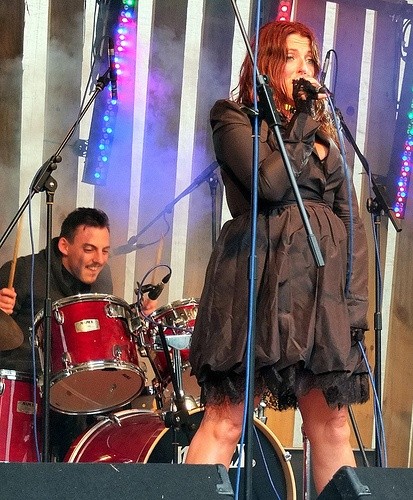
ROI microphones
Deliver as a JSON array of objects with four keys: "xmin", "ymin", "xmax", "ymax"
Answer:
[
  {"xmin": 148, "ymin": 273, "xmax": 171, "ymax": 300},
  {"xmin": 108, "ymin": 37, "xmax": 118, "ymax": 100},
  {"xmin": 319, "ymin": 51, "xmax": 330, "ymax": 85},
  {"xmin": 315, "ymin": 85, "xmax": 328, "ymax": 93}
]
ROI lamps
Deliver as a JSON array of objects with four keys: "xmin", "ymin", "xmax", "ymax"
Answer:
[
  {"xmin": 377, "ymin": 5, "xmax": 413, "ymax": 218},
  {"xmin": 76, "ymin": 1, "xmax": 137, "ymax": 185}
]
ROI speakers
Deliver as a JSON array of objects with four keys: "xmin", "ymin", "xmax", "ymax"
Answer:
[
  {"xmin": 285, "ymin": 447, "xmax": 413, "ymax": 500},
  {"xmin": 0, "ymin": 463, "xmax": 235, "ymax": 500}
]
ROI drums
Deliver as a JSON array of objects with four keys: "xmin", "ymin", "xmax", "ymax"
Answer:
[
  {"xmin": 61, "ymin": 405, "xmax": 297, "ymax": 500},
  {"xmin": 140, "ymin": 299, "xmax": 204, "ymax": 403},
  {"xmin": 0, "ymin": 366, "xmax": 46, "ymax": 463},
  {"xmin": 33, "ymin": 292, "xmax": 147, "ymax": 417}
]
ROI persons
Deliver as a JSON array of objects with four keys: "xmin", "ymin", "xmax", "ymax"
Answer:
[
  {"xmin": 187, "ymin": 22, "xmax": 371, "ymax": 493},
  {"xmin": 0, "ymin": 207, "xmax": 157, "ymax": 373}
]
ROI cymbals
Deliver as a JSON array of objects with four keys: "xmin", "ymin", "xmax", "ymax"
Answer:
[{"xmin": 0, "ymin": 308, "xmax": 24, "ymax": 351}]
